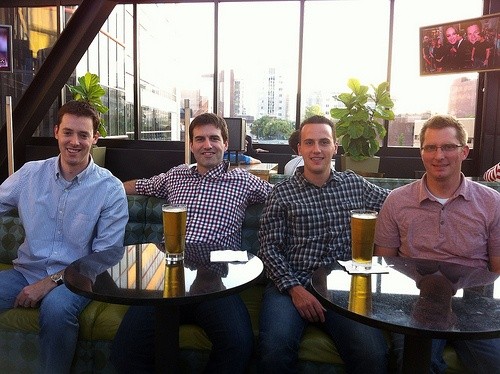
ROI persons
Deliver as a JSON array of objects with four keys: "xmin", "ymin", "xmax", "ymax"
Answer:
[
  {"xmin": 260, "ymin": 115, "xmax": 390, "ymax": 373},
  {"xmin": 64, "ymin": 248, "xmax": 126, "ymax": 292},
  {"xmin": 422, "ymin": 21, "xmax": 494, "ymax": 72},
  {"xmin": 223, "ymin": 130, "xmax": 261, "ymax": 164},
  {"xmin": 384, "ymin": 256, "xmax": 500, "ymax": 331},
  {"xmin": 0, "ymin": 102, "xmax": 129, "ymax": 374},
  {"xmin": 111, "ymin": 112, "xmax": 274, "ymax": 374},
  {"xmin": 284, "ymin": 130, "xmax": 304, "ymax": 176},
  {"xmin": 155, "ymin": 242, "xmax": 253, "ymax": 296},
  {"xmin": 374, "ymin": 118, "xmax": 500, "ymax": 374}
]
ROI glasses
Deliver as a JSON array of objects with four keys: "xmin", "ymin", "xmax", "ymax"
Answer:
[{"xmin": 421, "ymin": 144, "xmax": 463, "ymax": 153}]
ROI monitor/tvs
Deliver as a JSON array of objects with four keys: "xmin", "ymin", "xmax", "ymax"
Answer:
[{"xmin": 418, "ymin": 13, "xmax": 500, "ymax": 78}]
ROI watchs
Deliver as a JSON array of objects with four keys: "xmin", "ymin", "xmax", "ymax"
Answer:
[{"xmin": 50, "ymin": 273, "xmax": 64, "ymax": 286}]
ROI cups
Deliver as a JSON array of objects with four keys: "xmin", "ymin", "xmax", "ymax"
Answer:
[
  {"xmin": 351, "ymin": 209, "xmax": 378, "ymax": 264},
  {"xmin": 348, "ymin": 274, "xmax": 372, "ymax": 318},
  {"xmin": 163, "ymin": 260, "xmax": 185, "ymax": 298},
  {"xmin": 162, "ymin": 203, "xmax": 187, "ymax": 261}
]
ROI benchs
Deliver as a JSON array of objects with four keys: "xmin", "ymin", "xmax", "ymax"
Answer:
[{"xmin": 0, "ymin": 194, "xmax": 462, "ymax": 374}]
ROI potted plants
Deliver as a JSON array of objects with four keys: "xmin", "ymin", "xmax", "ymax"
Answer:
[{"xmin": 329, "ymin": 78, "xmax": 394, "ymax": 174}]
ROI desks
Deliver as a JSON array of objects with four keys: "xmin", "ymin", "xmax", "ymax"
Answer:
[
  {"xmin": 310, "ymin": 256, "xmax": 500, "ymax": 374},
  {"xmin": 64, "ymin": 244, "xmax": 265, "ymax": 374}
]
